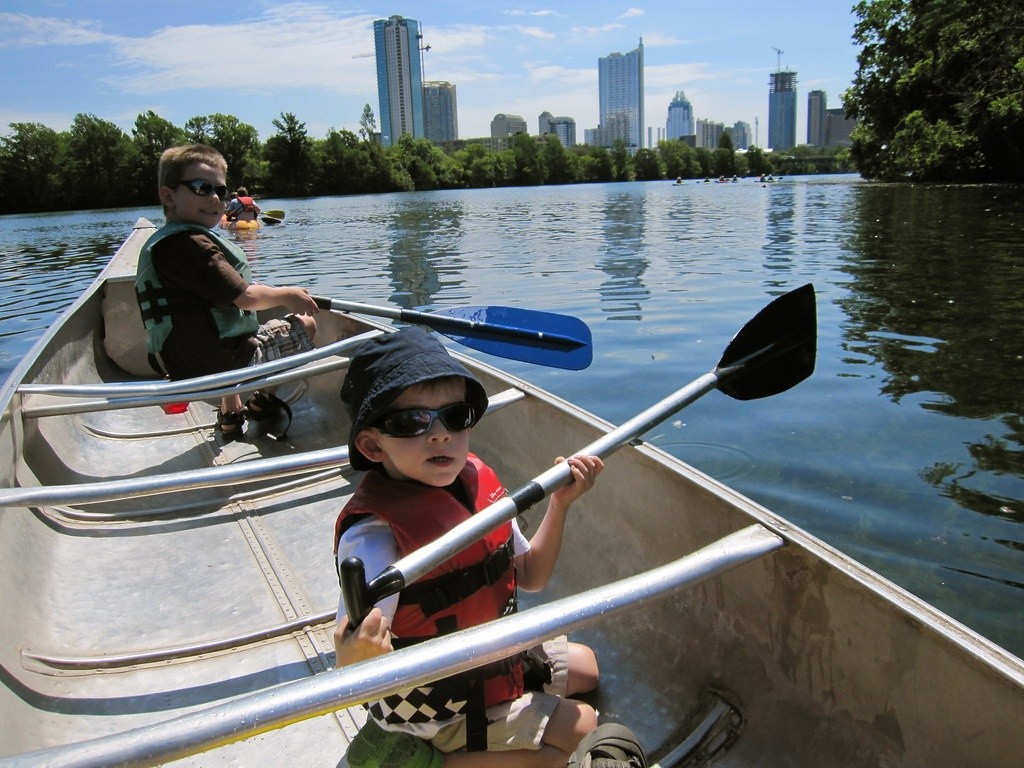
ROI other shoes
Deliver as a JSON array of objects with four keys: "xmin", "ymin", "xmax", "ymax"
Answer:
[{"xmin": 346, "ymin": 716, "xmax": 443, "ymax": 768}]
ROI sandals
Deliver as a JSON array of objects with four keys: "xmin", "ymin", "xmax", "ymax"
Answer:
[
  {"xmin": 244, "ymin": 389, "xmax": 278, "ymax": 421},
  {"xmin": 217, "ymin": 406, "xmax": 243, "ymax": 440}
]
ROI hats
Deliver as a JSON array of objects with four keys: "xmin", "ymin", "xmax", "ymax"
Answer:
[{"xmin": 339, "ymin": 327, "xmax": 488, "ymax": 471}]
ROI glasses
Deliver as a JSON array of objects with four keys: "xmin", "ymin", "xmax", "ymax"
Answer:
[
  {"xmin": 370, "ymin": 403, "xmax": 476, "ymax": 439},
  {"xmin": 177, "ymin": 178, "xmax": 229, "ymax": 200}
]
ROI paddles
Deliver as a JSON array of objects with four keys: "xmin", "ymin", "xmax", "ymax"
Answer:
[
  {"xmin": 308, "ymin": 292, "xmax": 595, "ymax": 374},
  {"xmin": 339, "ymin": 275, "xmax": 820, "ymax": 633},
  {"xmin": 259, "ymin": 209, "xmax": 285, "ymax": 219},
  {"xmin": 258, "ymin": 217, "xmax": 282, "ymax": 225}
]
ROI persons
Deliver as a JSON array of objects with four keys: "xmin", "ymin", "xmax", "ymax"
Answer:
[
  {"xmin": 733, "ymin": 175, "xmax": 737, "ymax": 181},
  {"xmin": 677, "ymin": 176, "xmax": 682, "ymax": 183},
  {"xmin": 768, "ymin": 174, "xmax": 774, "ymax": 180},
  {"xmin": 332, "ymin": 325, "xmax": 605, "ymax": 768},
  {"xmin": 225, "ymin": 186, "xmax": 261, "ymax": 220},
  {"xmin": 704, "ymin": 176, "xmax": 710, "ymax": 182},
  {"xmin": 719, "ymin": 175, "xmax": 725, "ymax": 181},
  {"xmin": 759, "ymin": 174, "xmax": 766, "ymax": 181},
  {"xmin": 134, "ymin": 145, "xmax": 320, "ymax": 441}
]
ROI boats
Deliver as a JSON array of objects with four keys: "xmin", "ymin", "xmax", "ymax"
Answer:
[{"xmin": 220, "ymin": 203, "xmax": 260, "ymax": 230}]
